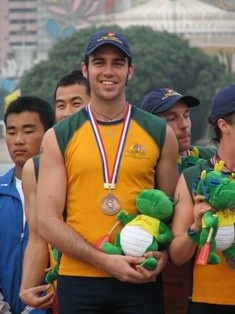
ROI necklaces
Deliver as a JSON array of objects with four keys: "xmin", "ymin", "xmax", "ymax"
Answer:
[{"xmin": 216, "ymin": 152, "xmax": 235, "ymax": 177}]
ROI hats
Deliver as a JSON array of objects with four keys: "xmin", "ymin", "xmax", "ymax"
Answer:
[
  {"xmin": 208, "ymin": 85, "xmax": 235, "ymax": 122},
  {"xmin": 140, "ymin": 87, "xmax": 201, "ymax": 114},
  {"xmin": 86, "ymin": 31, "xmax": 132, "ymax": 59}
]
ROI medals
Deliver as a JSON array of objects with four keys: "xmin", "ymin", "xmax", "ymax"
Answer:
[{"xmin": 100, "ymin": 194, "xmax": 121, "ymax": 216}]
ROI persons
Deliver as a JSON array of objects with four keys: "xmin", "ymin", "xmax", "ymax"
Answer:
[
  {"xmin": 35, "ymin": 29, "xmax": 180, "ymax": 314},
  {"xmin": 16, "ymin": 68, "xmax": 92, "ymax": 313},
  {"xmin": 0, "ymin": 94, "xmax": 58, "ymax": 313},
  {"xmin": 139, "ymin": 86, "xmax": 217, "ymax": 176},
  {"xmin": 168, "ymin": 83, "xmax": 235, "ymax": 313}
]
